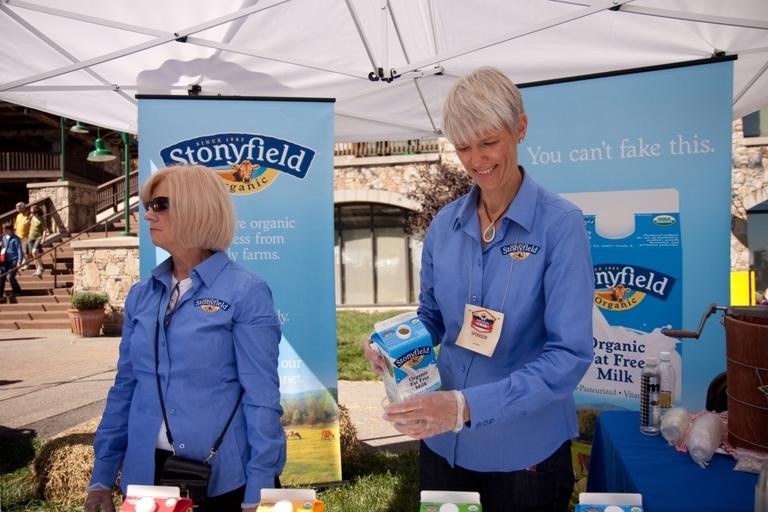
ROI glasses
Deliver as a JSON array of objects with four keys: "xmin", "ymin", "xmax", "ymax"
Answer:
[{"xmin": 146, "ymin": 195, "xmax": 169, "ymax": 212}]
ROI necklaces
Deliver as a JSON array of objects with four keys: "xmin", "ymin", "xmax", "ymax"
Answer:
[{"xmin": 481, "ymin": 201, "xmax": 512, "ymax": 245}]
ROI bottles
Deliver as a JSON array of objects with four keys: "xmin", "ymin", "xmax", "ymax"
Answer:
[
  {"xmin": 655, "ymin": 351, "xmax": 675, "ymax": 420},
  {"xmin": 640, "ymin": 357, "xmax": 662, "ymax": 438}
]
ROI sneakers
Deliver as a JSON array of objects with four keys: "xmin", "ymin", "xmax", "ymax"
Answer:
[{"xmin": 21, "ymin": 260, "xmax": 43, "ymax": 276}]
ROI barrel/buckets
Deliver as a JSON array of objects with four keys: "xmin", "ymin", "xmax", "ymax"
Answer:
[{"xmin": 720, "ymin": 312, "xmax": 768, "ymax": 453}]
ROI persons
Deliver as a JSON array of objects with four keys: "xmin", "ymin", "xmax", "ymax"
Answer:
[
  {"xmin": 1, "ymin": 223, "xmax": 23, "ymax": 298},
  {"xmin": 365, "ymin": 66, "xmax": 595, "ymax": 510},
  {"xmin": 13, "ymin": 202, "xmax": 30, "ymax": 271},
  {"xmin": 84, "ymin": 164, "xmax": 286, "ymax": 512},
  {"xmin": 26, "ymin": 205, "xmax": 46, "ymax": 276}
]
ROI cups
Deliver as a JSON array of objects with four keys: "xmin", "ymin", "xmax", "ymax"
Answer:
[
  {"xmin": 660, "ymin": 407, "xmax": 723, "ymax": 464},
  {"xmin": 381, "ymin": 389, "xmax": 419, "ymax": 427}
]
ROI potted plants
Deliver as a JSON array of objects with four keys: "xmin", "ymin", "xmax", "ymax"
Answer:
[{"xmin": 68, "ymin": 292, "xmax": 110, "ymax": 337}]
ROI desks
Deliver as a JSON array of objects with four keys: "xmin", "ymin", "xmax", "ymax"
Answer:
[{"xmin": 585, "ymin": 411, "xmax": 760, "ymax": 512}]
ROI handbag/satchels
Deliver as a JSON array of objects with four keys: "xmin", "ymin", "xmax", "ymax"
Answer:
[
  {"xmin": 161, "ymin": 454, "xmax": 213, "ymax": 506},
  {"xmin": 1, "ymin": 252, "xmax": 7, "ymax": 263}
]
